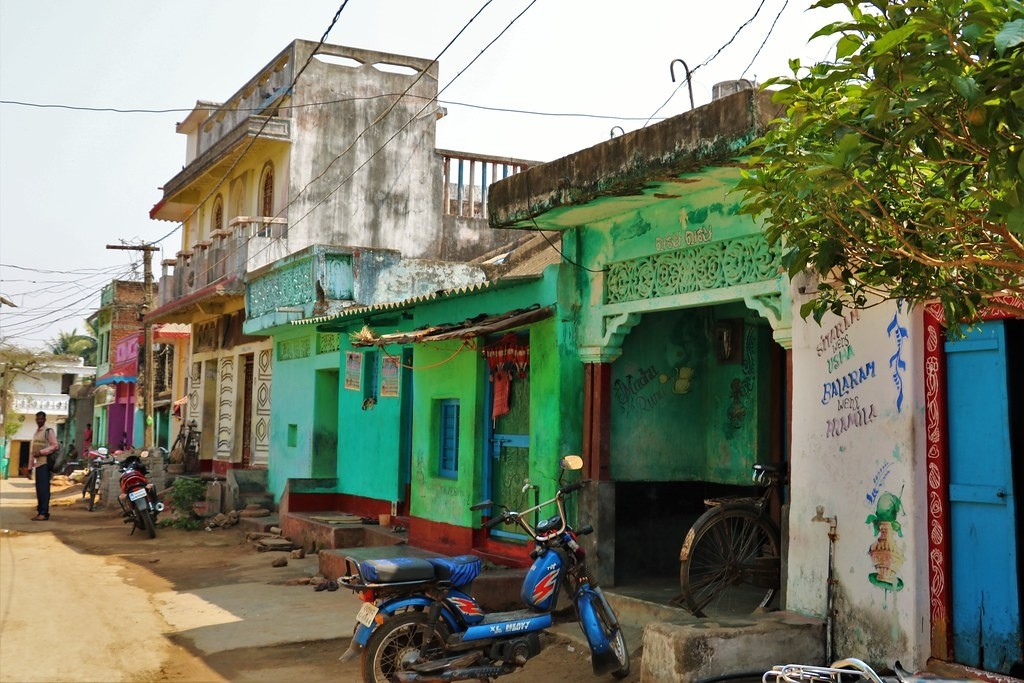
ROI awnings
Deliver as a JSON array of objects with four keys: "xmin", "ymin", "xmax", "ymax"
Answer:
[
  {"xmin": 138, "ymin": 322, "xmax": 192, "ymax": 346},
  {"xmin": 97, "ymin": 360, "xmax": 139, "ymax": 385}
]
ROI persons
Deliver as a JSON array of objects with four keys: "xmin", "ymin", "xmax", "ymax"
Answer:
[
  {"xmin": 60, "ymin": 424, "xmax": 93, "ymax": 475},
  {"xmin": 28, "ymin": 412, "xmax": 60, "ymax": 521}
]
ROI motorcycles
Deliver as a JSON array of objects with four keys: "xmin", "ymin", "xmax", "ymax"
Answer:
[
  {"xmin": 338, "ymin": 455, "xmax": 630, "ymax": 683},
  {"xmin": 764, "ymin": 658, "xmax": 976, "ymax": 683},
  {"xmin": 84, "ymin": 430, "xmax": 135, "ymax": 470},
  {"xmin": 98, "ymin": 446, "xmax": 164, "ymax": 539}
]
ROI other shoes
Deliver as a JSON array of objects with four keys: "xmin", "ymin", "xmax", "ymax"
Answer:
[{"xmin": 32, "ymin": 515, "xmax": 48, "ymax": 521}]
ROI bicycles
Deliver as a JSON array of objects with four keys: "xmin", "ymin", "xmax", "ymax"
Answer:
[
  {"xmin": 168, "ymin": 413, "xmax": 201, "ymax": 474},
  {"xmin": 664, "ymin": 463, "xmax": 785, "ymax": 618},
  {"xmin": 82, "ymin": 456, "xmax": 102, "ymax": 512}
]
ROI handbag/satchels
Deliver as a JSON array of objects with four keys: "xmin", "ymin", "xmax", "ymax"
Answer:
[{"xmin": 46, "ymin": 428, "xmax": 67, "ymax": 473}]
